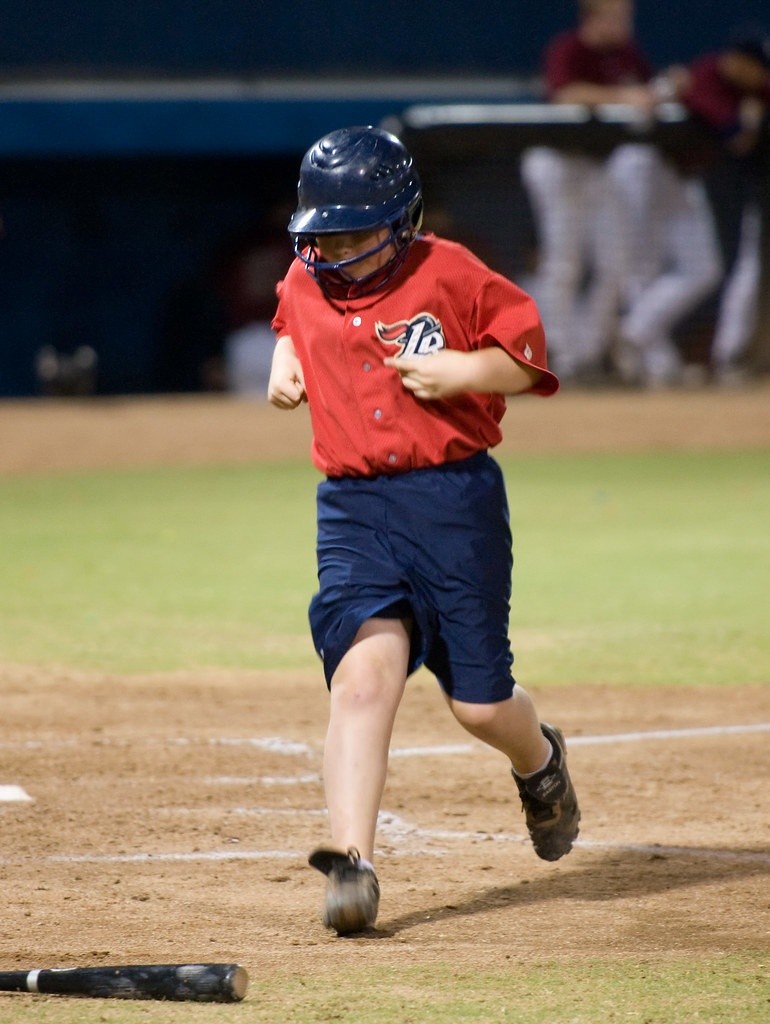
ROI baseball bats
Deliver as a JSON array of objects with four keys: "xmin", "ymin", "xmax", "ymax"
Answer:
[{"xmin": 0, "ymin": 961, "xmax": 252, "ymax": 1004}]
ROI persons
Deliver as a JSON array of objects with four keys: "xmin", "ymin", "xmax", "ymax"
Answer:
[
  {"xmin": 519, "ymin": 2, "xmax": 770, "ymax": 389},
  {"xmin": 265, "ymin": 126, "xmax": 580, "ymax": 933}
]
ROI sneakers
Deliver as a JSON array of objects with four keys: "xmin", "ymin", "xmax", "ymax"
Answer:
[
  {"xmin": 308, "ymin": 850, "xmax": 379, "ymax": 931},
  {"xmin": 510, "ymin": 723, "xmax": 581, "ymax": 861}
]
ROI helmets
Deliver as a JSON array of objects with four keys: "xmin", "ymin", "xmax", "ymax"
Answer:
[{"xmin": 287, "ymin": 126, "xmax": 424, "ymax": 234}]
